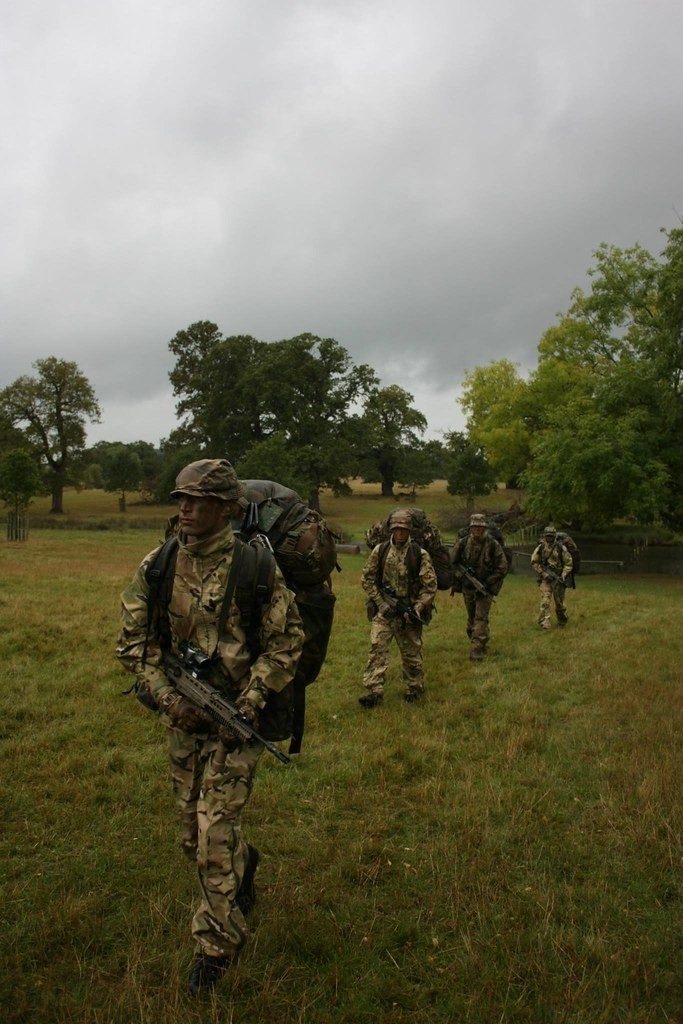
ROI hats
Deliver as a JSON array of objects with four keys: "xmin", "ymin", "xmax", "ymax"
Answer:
[
  {"xmin": 468, "ymin": 513, "xmax": 489, "ymax": 528},
  {"xmin": 389, "ymin": 511, "xmax": 414, "ymax": 531},
  {"xmin": 543, "ymin": 526, "xmax": 557, "ymax": 537},
  {"xmin": 169, "ymin": 459, "xmax": 248, "ymax": 501}
]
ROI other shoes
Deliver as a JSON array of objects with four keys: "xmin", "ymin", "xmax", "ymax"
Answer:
[
  {"xmin": 189, "ymin": 953, "xmax": 233, "ymax": 999},
  {"xmin": 404, "ymin": 694, "xmax": 419, "ymax": 702},
  {"xmin": 470, "ymin": 650, "xmax": 483, "ymax": 661},
  {"xmin": 236, "ymin": 844, "xmax": 259, "ymax": 916},
  {"xmin": 358, "ymin": 693, "xmax": 384, "ymax": 707}
]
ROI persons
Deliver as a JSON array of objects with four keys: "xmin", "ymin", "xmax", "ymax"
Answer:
[
  {"xmin": 116, "ymin": 458, "xmax": 304, "ymax": 990},
  {"xmin": 449, "ymin": 513, "xmax": 508, "ymax": 662},
  {"xmin": 358, "ymin": 510, "xmax": 439, "ymax": 707},
  {"xmin": 530, "ymin": 527, "xmax": 573, "ymax": 629}
]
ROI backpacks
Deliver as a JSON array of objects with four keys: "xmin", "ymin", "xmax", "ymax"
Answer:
[
  {"xmin": 455, "ymin": 519, "xmax": 513, "ymax": 583},
  {"xmin": 364, "ymin": 508, "xmax": 453, "ymax": 591},
  {"xmin": 537, "ymin": 532, "xmax": 582, "ymax": 590},
  {"xmin": 142, "ymin": 480, "xmax": 343, "ymax": 758}
]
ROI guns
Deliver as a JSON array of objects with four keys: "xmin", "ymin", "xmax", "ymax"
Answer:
[
  {"xmin": 376, "ymin": 584, "xmax": 427, "ymax": 625},
  {"xmin": 160, "ymin": 637, "xmax": 292, "ymax": 766},
  {"xmin": 455, "ymin": 561, "xmax": 497, "ymax": 604},
  {"xmin": 541, "ymin": 564, "xmax": 570, "ymax": 588}
]
